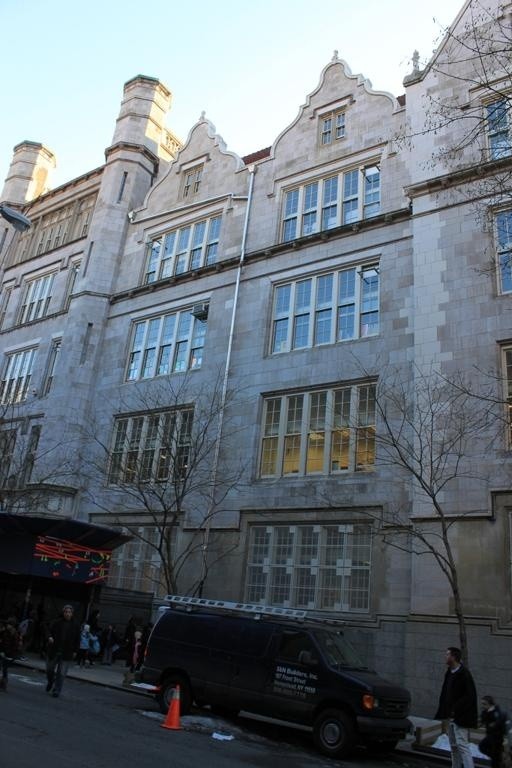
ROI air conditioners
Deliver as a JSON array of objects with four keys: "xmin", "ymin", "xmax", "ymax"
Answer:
[{"xmin": 191, "ymin": 303, "xmax": 206, "ymax": 316}]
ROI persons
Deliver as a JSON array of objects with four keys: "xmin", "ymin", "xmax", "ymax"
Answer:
[
  {"xmin": 0, "ymin": 603, "xmax": 154, "ymax": 697},
  {"xmin": 478, "ymin": 695, "xmax": 507, "ymax": 768},
  {"xmin": 433, "ymin": 646, "xmax": 478, "ymax": 768}
]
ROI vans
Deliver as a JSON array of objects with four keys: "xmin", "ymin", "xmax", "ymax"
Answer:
[{"xmin": 134, "ymin": 596, "xmax": 415, "ymax": 759}]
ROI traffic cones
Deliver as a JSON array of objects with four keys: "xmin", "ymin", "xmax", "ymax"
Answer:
[{"xmin": 160, "ymin": 682, "xmax": 185, "ymax": 731}]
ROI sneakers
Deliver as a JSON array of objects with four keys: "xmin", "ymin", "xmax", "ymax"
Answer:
[
  {"xmin": 45, "ymin": 678, "xmax": 55, "ymax": 692},
  {"xmin": 52, "ymin": 685, "xmax": 61, "ymax": 698}
]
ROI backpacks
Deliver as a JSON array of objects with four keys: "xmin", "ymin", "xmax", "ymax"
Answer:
[{"xmin": 88, "ymin": 639, "xmax": 100, "ymax": 656}]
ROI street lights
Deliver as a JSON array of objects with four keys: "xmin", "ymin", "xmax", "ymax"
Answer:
[{"xmin": 0, "ymin": 205, "xmax": 31, "ymax": 234}]
